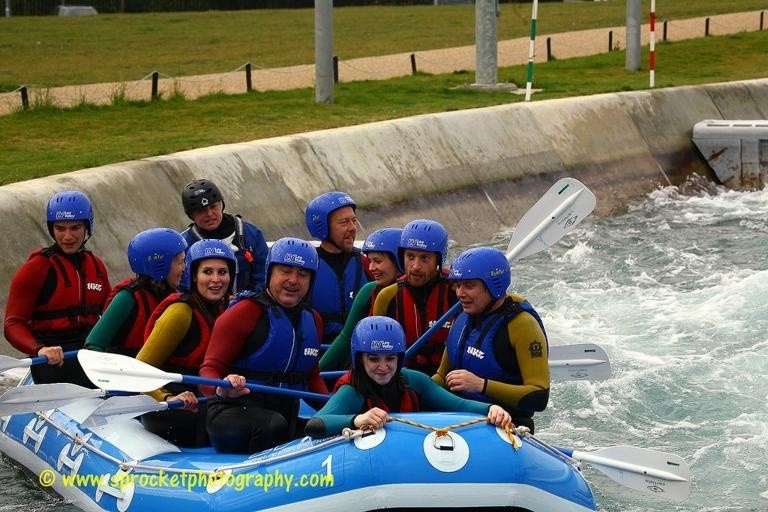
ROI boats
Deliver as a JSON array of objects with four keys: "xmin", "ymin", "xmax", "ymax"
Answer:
[{"xmin": 1, "ymin": 355, "xmax": 598, "ymax": 512}]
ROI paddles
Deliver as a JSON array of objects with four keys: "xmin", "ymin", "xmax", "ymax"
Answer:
[
  {"xmin": 404, "ymin": 177, "xmax": 595, "ymax": 357},
  {"xmin": 80, "ymin": 396, "xmax": 220, "ymax": 427},
  {"xmin": 552, "ymin": 443, "xmax": 690, "ymax": 502},
  {"xmin": 0, "ymin": 384, "xmax": 139, "ymax": 417},
  {"xmin": 320, "ymin": 346, "xmax": 612, "ymax": 382},
  {"xmin": 0, "ymin": 351, "xmax": 77, "ymax": 375},
  {"xmin": 78, "ymin": 348, "xmax": 332, "ymax": 402}
]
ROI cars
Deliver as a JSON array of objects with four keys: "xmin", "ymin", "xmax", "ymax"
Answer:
[{"xmin": 54, "ymin": 5, "xmax": 99, "ymax": 17}]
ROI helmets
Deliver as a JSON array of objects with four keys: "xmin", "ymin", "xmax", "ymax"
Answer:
[
  {"xmin": 264, "ymin": 237, "xmax": 318, "ymax": 285},
  {"xmin": 351, "ymin": 315, "xmax": 407, "ymax": 370},
  {"xmin": 447, "ymin": 247, "xmax": 513, "ymax": 300},
  {"xmin": 46, "ymin": 191, "xmax": 93, "ymax": 244},
  {"xmin": 304, "ymin": 190, "xmax": 356, "ymax": 241},
  {"xmin": 127, "ymin": 227, "xmax": 188, "ymax": 281},
  {"xmin": 180, "ymin": 178, "xmax": 223, "ymax": 213},
  {"xmin": 397, "ymin": 218, "xmax": 448, "ymax": 275},
  {"xmin": 184, "ymin": 238, "xmax": 236, "ymax": 292},
  {"xmin": 363, "ymin": 228, "xmax": 402, "ymax": 272}
]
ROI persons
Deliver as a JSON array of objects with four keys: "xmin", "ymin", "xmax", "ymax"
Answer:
[
  {"xmin": 179, "ymin": 178, "xmax": 270, "ymax": 294},
  {"xmin": 369, "ymin": 218, "xmax": 465, "ymax": 376},
  {"xmin": 429, "ymin": 246, "xmax": 551, "ymax": 436},
  {"xmin": 133, "ymin": 238, "xmax": 239, "ymax": 448},
  {"xmin": 3, "ymin": 188, "xmax": 114, "ymax": 390},
  {"xmin": 304, "ymin": 190, "xmax": 373, "ymax": 344},
  {"xmin": 195, "ymin": 236, "xmax": 333, "ymax": 454},
  {"xmin": 81, "ymin": 227, "xmax": 190, "ymax": 399},
  {"xmin": 305, "ymin": 314, "xmax": 516, "ymax": 440},
  {"xmin": 319, "ymin": 227, "xmax": 405, "ymax": 372}
]
